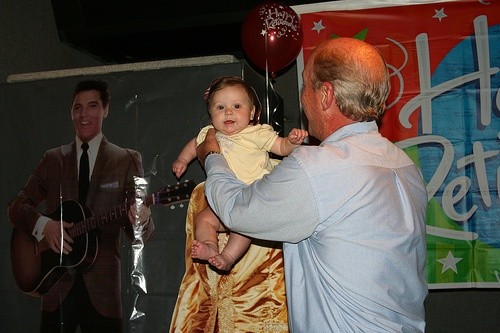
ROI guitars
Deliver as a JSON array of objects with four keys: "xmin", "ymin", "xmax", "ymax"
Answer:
[{"xmin": 7, "ymin": 178, "xmax": 196, "ymax": 299}]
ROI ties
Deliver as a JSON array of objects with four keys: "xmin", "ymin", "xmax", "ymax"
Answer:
[{"xmin": 78, "ymin": 142, "xmax": 90, "ymax": 204}]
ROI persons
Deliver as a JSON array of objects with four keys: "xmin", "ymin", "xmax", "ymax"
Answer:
[
  {"xmin": 195, "ymin": 37, "xmax": 429, "ymax": 333},
  {"xmin": 7, "ymin": 80, "xmax": 154, "ymax": 333},
  {"xmin": 171, "ymin": 160, "xmax": 290, "ymax": 333},
  {"xmin": 172, "ymin": 75, "xmax": 309, "ymax": 271}
]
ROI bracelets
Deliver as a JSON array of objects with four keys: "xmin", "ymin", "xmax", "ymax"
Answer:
[{"xmin": 203, "ymin": 151, "xmax": 221, "ymax": 159}]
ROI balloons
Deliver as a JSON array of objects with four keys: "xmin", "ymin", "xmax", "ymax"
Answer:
[{"xmin": 241, "ymin": 1, "xmax": 303, "ymax": 75}]
